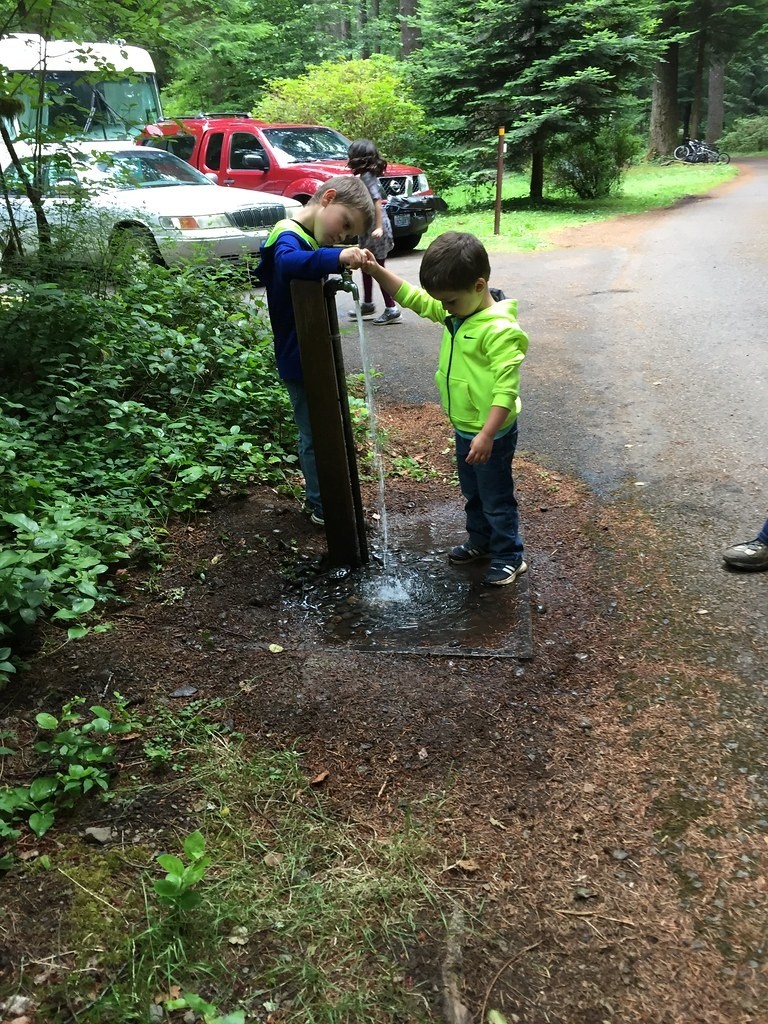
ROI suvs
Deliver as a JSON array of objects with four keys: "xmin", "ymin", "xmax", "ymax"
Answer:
[{"xmin": 133, "ymin": 116, "xmax": 447, "ymax": 253}]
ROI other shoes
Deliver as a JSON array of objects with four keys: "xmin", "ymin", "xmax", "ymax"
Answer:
[
  {"xmin": 311, "ymin": 512, "xmax": 325, "ymax": 526},
  {"xmin": 302, "ymin": 499, "xmax": 317, "ymax": 514}
]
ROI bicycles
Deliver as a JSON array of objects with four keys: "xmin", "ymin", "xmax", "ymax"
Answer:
[{"xmin": 673, "ymin": 136, "xmax": 731, "ymax": 165}]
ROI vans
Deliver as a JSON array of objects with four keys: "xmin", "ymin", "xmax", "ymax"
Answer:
[{"xmin": 1, "ymin": 30, "xmax": 163, "ymax": 185}]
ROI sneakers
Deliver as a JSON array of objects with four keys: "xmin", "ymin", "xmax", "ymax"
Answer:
[
  {"xmin": 449, "ymin": 537, "xmax": 492, "ymax": 565},
  {"xmin": 347, "ymin": 303, "xmax": 378, "ymax": 322},
  {"xmin": 722, "ymin": 538, "xmax": 768, "ymax": 570},
  {"xmin": 486, "ymin": 560, "xmax": 527, "ymax": 585},
  {"xmin": 372, "ymin": 306, "xmax": 404, "ymax": 325}
]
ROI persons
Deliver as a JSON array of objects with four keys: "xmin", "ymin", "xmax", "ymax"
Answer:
[
  {"xmin": 718, "ymin": 517, "xmax": 768, "ymax": 574},
  {"xmin": 359, "ymin": 229, "xmax": 533, "ymax": 586},
  {"xmin": 250, "ymin": 173, "xmax": 379, "ymax": 527},
  {"xmin": 343, "ymin": 137, "xmax": 406, "ymax": 327}
]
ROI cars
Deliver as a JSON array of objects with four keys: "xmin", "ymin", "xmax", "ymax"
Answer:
[{"xmin": 1, "ymin": 141, "xmax": 304, "ymax": 296}]
